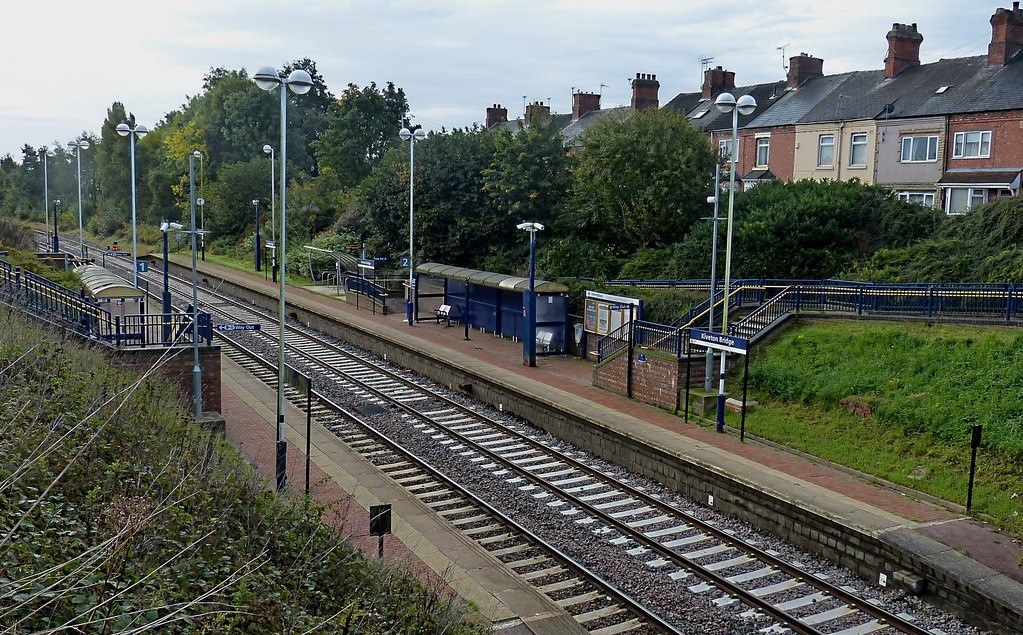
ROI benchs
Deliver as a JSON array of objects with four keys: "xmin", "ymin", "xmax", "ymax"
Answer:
[
  {"xmin": 432, "ymin": 304, "xmax": 454, "ymax": 327},
  {"xmin": 535, "ymin": 330, "xmax": 554, "ymax": 352}
]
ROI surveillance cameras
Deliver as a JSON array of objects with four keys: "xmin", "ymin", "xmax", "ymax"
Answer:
[
  {"xmin": 160, "ymin": 222, "xmax": 183, "ymax": 231},
  {"xmin": 707, "ymin": 196, "xmax": 716, "ymax": 203},
  {"xmin": 516, "ymin": 221, "xmax": 545, "ymax": 230}
]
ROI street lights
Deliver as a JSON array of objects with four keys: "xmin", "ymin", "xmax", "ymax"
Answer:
[
  {"xmin": 399, "ymin": 128, "xmax": 426, "ymax": 325},
  {"xmin": 45, "ymin": 150, "xmax": 56, "ymax": 243},
  {"xmin": 115, "ymin": 123, "xmax": 149, "ymax": 290},
  {"xmin": 67, "ymin": 140, "xmax": 90, "ymax": 259},
  {"xmin": 714, "ymin": 90, "xmax": 759, "ymax": 433},
  {"xmin": 252, "ymin": 64, "xmax": 313, "ymax": 493},
  {"xmin": 194, "ymin": 150, "xmax": 206, "ymax": 263},
  {"xmin": 263, "ymin": 144, "xmax": 277, "ymax": 283}
]
